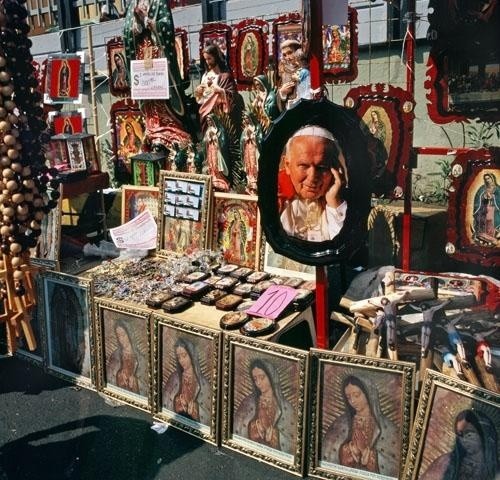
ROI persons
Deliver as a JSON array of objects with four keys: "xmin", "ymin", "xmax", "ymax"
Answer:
[
  {"xmin": 194, "ymin": 43, "xmax": 244, "ymax": 142},
  {"xmin": 418, "ymin": 408, "xmax": 500, "ymax": 480},
  {"xmin": 123, "ymin": 0, "xmax": 199, "ymax": 153},
  {"xmin": 61, "ymin": 118, "xmax": 74, "ymax": 133},
  {"xmin": 239, "ymin": 111, "xmax": 263, "ymax": 194},
  {"xmin": 104, "ymin": 320, "xmax": 149, "ymax": 400},
  {"xmin": 279, "ymin": 39, "xmax": 313, "ymax": 110},
  {"xmin": 251, "ymin": 74, "xmax": 274, "ymax": 134},
  {"xmin": 98, "ymin": 0, "xmax": 121, "ymax": 23},
  {"xmin": 364, "ymin": 108, "xmax": 388, "ymax": 171},
  {"xmin": 57, "ymin": 59, "xmax": 71, "ymax": 95},
  {"xmin": 112, "ymin": 52, "xmax": 130, "ymax": 87},
  {"xmin": 243, "ymin": 34, "xmax": 257, "ymax": 78},
  {"xmin": 473, "ymin": 171, "xmax": 500, "ymax": 245},
  {"xmin": 232, "ymin": 357, "xmax": 296, "ymax": 455},
  {"xmin": 274, "ymin": 127, "xmax": 349, "ymax": 243},
  {"xmin": 224, "ymin": 208, "xmax": 248, "ymax": 261},
  {"xmin": 121, "ymin": 120, "xmax": 144, "ymax": 164},
  {"xmin": 203, "ymin": 114, "xmax": 234, "ymax": 188},
  {"xmin": 321, "ymin": 372, "xmax": 399, "ymax": 477},
  {"xmin": 132, "ymin": 196, "xmax": 147, "ymax": 216},
  {"xmin": 161, "ymin": 337, "xmax": 212, "ymax": 427}
]
